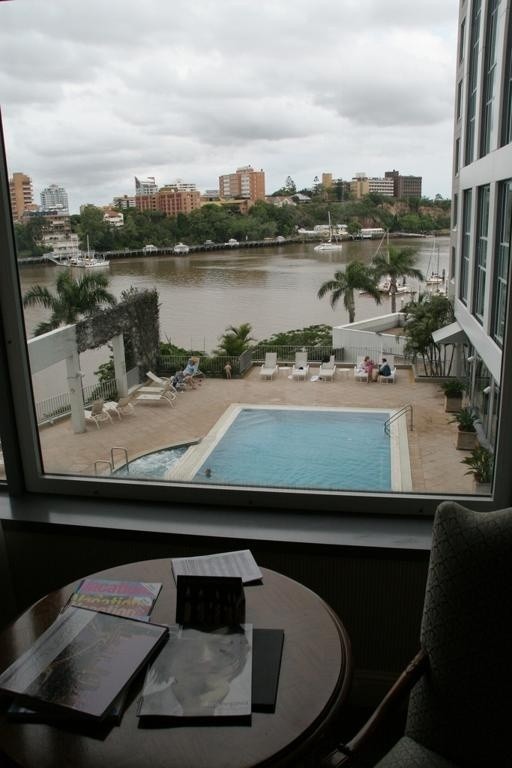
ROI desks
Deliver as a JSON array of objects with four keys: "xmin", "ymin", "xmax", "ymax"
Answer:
[{"xmin": 2, "ymin": 556, "xmax": 353, "ymax": 767}]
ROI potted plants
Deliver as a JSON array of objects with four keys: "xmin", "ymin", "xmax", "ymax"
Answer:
[{"xmin": 437, "ymin": 374, "xmax": 496, "ymax": 494}]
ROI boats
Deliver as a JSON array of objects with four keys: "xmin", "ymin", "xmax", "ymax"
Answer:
[
  {"xmin": 423, "ymin": 233, "xmax": 445, "ymax": 286},
  {"xmin": 313, "ymin": 211, "xmax": 343, "ymax": 253},
  {"xmin": 64, "ymin": 234, "xmax": 110, "ymax": 269},
  {"xmin": 359, "ymin": 226, "xmax": 410, "ymax": 296}
]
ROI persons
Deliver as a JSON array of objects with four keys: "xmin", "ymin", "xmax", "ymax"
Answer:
[
  {"xmin": 172, "ymin": 357, "xmax": 197, "ymax": 392},
  {"xmin": 360, "ymin": 356, "xmax": 391, "ymax": 382},
  {"xmin": 224, "ymin": 360, "xmax": 232, "ymax": 379}
]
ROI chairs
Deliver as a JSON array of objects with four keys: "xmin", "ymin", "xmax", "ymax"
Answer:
[
  {"xmin": 257, "ymin": 352, "xmax": 399, "ymax": 383},
  {"xmin": 330, "ymin": 495, "xmax": 510, "ymax": 767},
  {"xmin": 83, "ymin": 355, "xmax": 205, "ymax": 426}
]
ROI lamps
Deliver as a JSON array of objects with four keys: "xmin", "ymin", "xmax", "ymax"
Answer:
[{"xmin": 462, "ymin": 350, "xmax": 499, "ymax": 398}]
